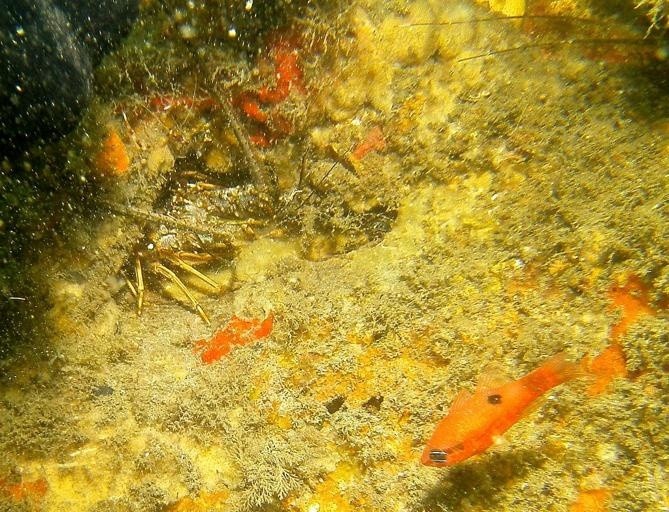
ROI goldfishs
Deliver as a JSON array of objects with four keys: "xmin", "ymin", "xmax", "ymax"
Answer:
[{"xmin": 421, "ymin": 351, "xmax": 584, "ymax": 469}]
